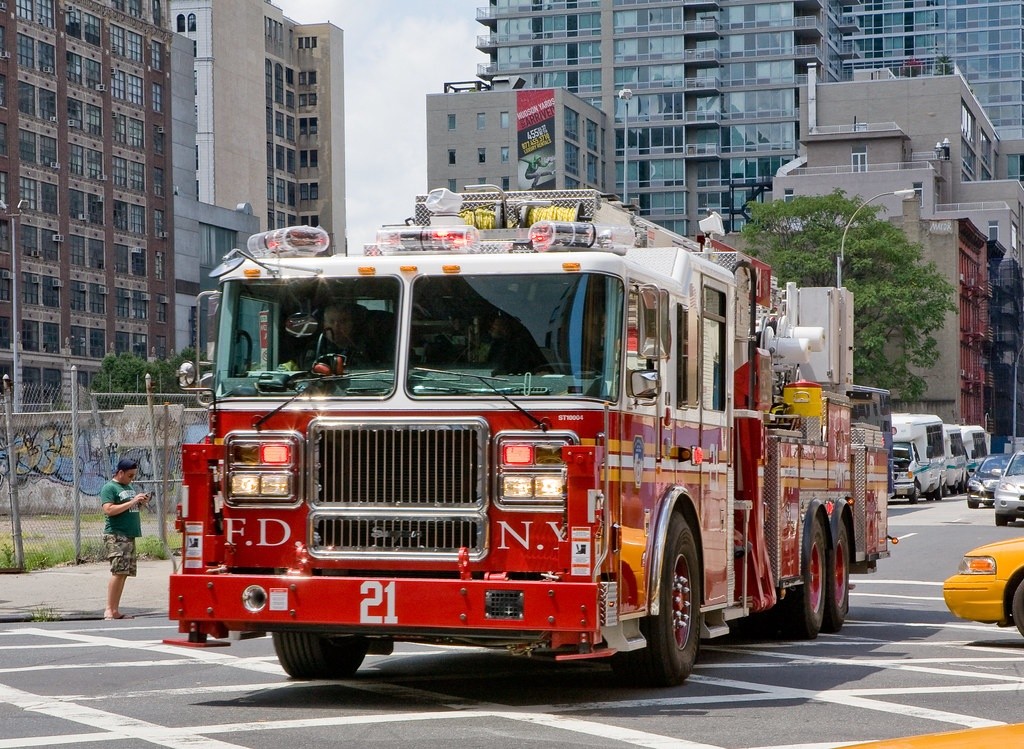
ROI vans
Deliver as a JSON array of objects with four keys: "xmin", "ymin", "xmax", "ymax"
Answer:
[
  {"xmin": 891, "ymin": 412, "xmax": 947, "ymax": 505},
  {"xmin": 941, "ymin": 424, "xmax": 968, "ymax": 497},
  {"xmin": 959, "ymin": 426, "xmax": 989, "ymax": 482}
]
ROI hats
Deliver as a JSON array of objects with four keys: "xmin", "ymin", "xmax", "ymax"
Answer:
[{"xmin": 118, "ymin": 460, "xmax": 137, "ymax": 470}]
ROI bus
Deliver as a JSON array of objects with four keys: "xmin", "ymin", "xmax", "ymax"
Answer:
[{"xmin": 848, "ymin": 385, "xmax": 895, "ymax": 500}]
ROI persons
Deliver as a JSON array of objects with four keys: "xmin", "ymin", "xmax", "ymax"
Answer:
[
  {"xmin": 520, "ymin": 156, "xmax": 555, "ymax": 189},
  {"xmin": 100, "ymin": 459, "xmax": 148, "ymax": 620},
  {"xmin": 286, "ymin": 304, "xmax": 386, "ymax": 371}
]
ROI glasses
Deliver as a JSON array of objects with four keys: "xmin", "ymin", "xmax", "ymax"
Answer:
[{"xmin": 124, "ymin": 472, "xmax": 135, "ymax": 478}]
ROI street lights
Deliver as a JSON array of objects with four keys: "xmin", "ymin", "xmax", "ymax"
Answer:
[
  {"xmin": 0, "ymin": 199, "xmax": 30, "ymax": 413},
  {"xmin": 836, "ymin": 188, "xmax": 916, "ymax": 287},
  {"xmin": 619, "ymin": 88, "xmax": 633, "ymax": 207}
]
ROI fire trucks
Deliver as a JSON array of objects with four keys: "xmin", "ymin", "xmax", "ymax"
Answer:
[{"xmin": 162, "ymin": 184, "xmax": 900, "ymax": 688}]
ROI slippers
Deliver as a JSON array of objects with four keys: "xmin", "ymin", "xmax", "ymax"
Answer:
[
  {"xmin": 105, "ymin": 617, "xmax": 114, "ymax": 620},
  {"xmin": 114, "ymin": 615, "xmax": 134, "ymax": 619}
]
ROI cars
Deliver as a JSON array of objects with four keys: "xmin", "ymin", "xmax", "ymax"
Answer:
[
  {"xmin": 967, "ymin": 452, "xmax": 1014, "ymax": 509},
  {"xmin": 942, "ymin": 537, "xmax": 1023, "ymax": 641},
  {"xmin": 990, "ymin": 451, "xmax": 1024, "ymax": 527}
]
